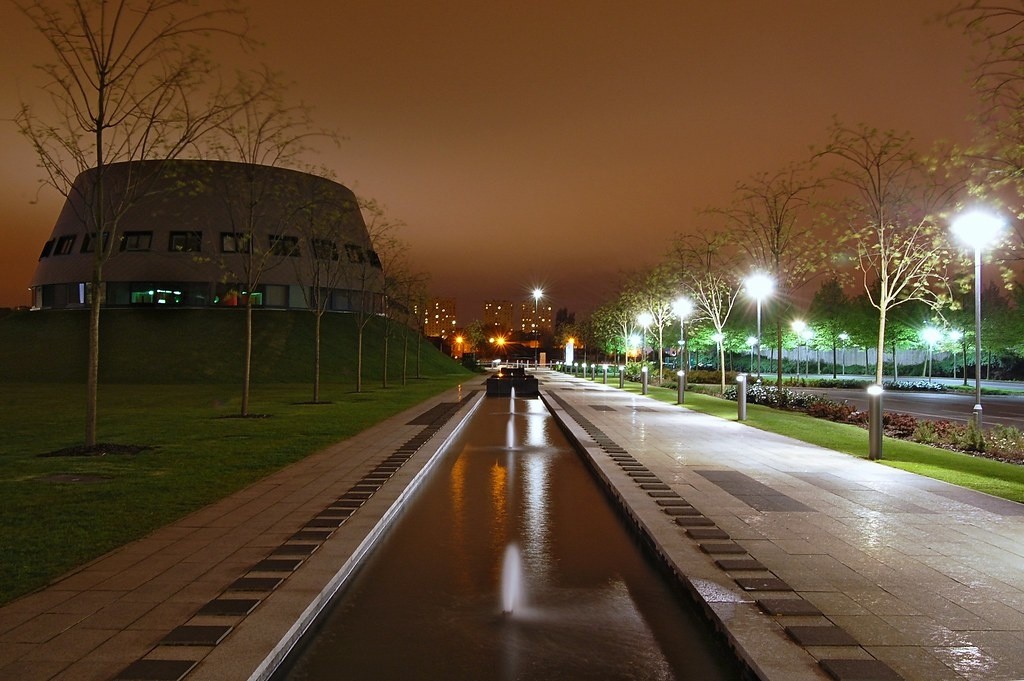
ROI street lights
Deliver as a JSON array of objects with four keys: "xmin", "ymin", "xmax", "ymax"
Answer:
[
  {"xmin": 948, "ymin": 330, "xmax": 960, "ymax": 379},
  {"xmin": 639, "ymin": 310, "xmax": 653, "ymax": 362},
  {"xmin": 792, "ymin": 320, "xmax": 803, "ymax": 378},
  {"xmin": 713, "ymin": 335, "xmax": 722, "ymax": 373},
  {"xmin": 745, "ymin": 337, "xmax": 758, "ymax": 374},
  {"xmin": 923, "ymin": 328, "xmax": 939, "ymax": 383},
  {"xmin": 673, "ymin": 297, "xmax": 690, "ymax": 373},
  {"xmin": 803, "ymin": 332, "xmax": 812, "ymax": 380},
  {"xmin": 745, "ymin": 274, "xmax": 772, "ymax": 385},
  {"xmin": 950, "ymin": 211, "xmax": 997, "ymax": 441},
  {"xmin": 839, "ymin": 333, "xmax": 848, "ymax": 378},
  {"xmin": 532, "ymin": 288, "xmax": 543, "ymax": 364}
]
